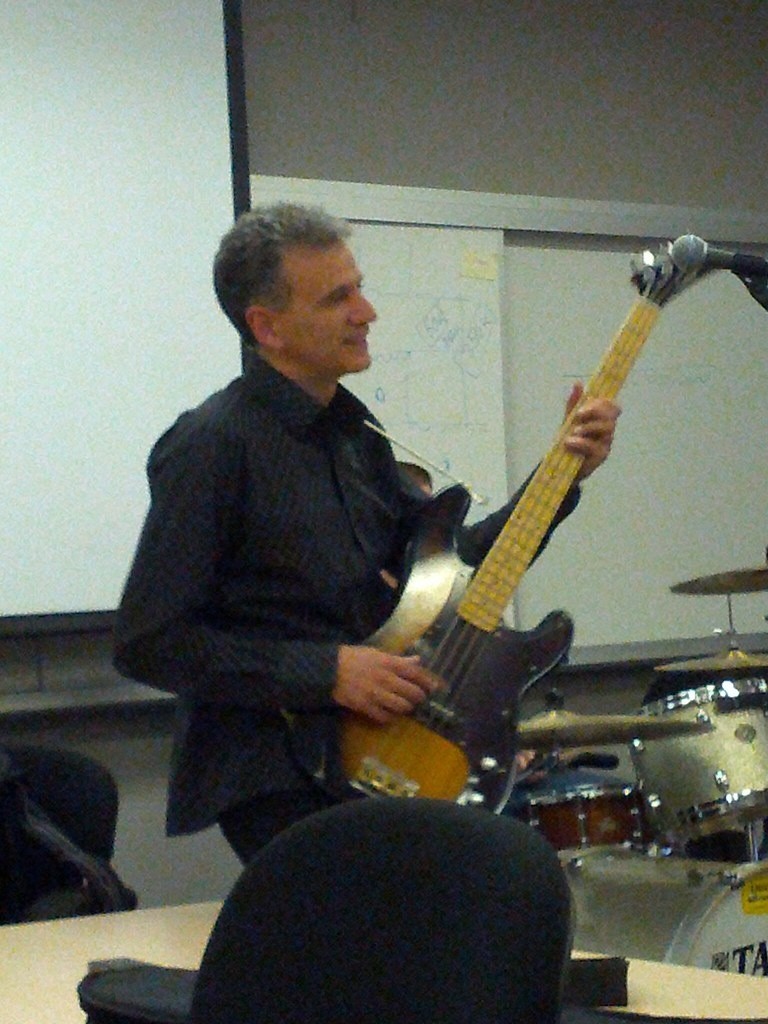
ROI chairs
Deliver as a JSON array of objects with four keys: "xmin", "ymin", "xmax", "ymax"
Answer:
[
  {"xmin": 76, "ymin": 799, "xmax": 575, "ymax": 1024},
  {"xmin": 1, "ymin": 746, "xmax": 138, "ymax": 928}
]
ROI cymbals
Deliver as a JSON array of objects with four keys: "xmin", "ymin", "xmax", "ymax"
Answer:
[
  {"xmin": 674, "ymin": 567, "xmax": 768, "ymax": 598},
  {"xmin": 656, "ymin": 652, "xmax": 768, "ymax": 670},
  {"xmin": 511, "ymin": 709, "xmax": 713, "ymax": 745}
]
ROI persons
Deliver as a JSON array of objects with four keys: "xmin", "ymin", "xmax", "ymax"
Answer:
[{"xmin": 109, "ymin": 200, "xmax": 624, "ymax": 863}]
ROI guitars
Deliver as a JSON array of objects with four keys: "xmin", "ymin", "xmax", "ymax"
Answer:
[{"xmin": 308, "ymin": 252, "xmax": 687, "ymax": 810}]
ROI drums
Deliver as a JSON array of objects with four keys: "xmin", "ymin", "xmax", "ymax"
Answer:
[
  {"xmin": 617, "ymin": 678, "xmax": 768, "ymax": 841},
  {"xmin": 505, "ymin": 768, "xmax": 646, "ymax": 848},
  {"xmin": 561, "ymin": 845, "xmax": 768, "ymax": 979}
]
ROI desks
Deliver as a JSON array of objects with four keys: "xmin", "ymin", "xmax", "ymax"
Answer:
[{"xmin": 2, "ymin": 900, "xmax": 768, "ymax": 1024}]
